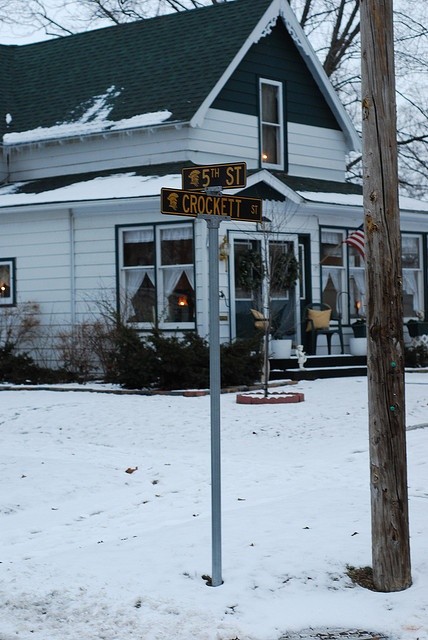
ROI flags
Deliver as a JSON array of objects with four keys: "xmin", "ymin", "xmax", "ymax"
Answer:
[{"xmin": 341, "ymin": 222, "xmax": 365, "ymax": 259}]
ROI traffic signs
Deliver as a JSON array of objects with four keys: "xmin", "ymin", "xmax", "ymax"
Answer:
[
  {"xmin": 182, "ymin": 162, "xmax": 246, "ymax": 191},
  {"xmin": 160, "ymin": 187, "xmax": 262, "ymax": 223}
]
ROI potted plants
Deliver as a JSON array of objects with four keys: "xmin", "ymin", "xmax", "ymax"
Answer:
[{"xmin": 270, "ymin": 329, "xmax": 296, "ymax": 359}]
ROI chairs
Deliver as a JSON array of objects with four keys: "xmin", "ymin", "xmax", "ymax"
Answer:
[{"xmin": 303, "ymin": 303, "xmax": 345, "ymax": 355}]
